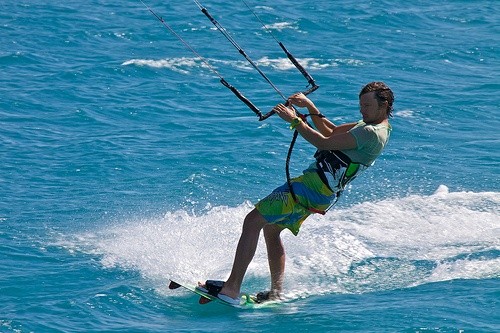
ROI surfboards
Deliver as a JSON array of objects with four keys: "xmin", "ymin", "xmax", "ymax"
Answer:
[{"xmin": 166, "ymin": 275, "xmax": 298, "ymax": 305}]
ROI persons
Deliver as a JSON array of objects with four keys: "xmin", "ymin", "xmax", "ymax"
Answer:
[{"xmin": 197, "ymin": 81, "xmax": 395, "ymax": 303}]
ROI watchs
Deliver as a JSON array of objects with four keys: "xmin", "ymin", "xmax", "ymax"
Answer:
[{"xmin": 289, "ymin": 116, "xmax": 302, "ymax": 130}]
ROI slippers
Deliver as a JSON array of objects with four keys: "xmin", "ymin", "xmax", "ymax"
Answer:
[
  {"xmin": 247, "ymin": 291, "xmax": 285, "ymax": 304},
  {"xmin": 195, "ymin": 279, "xmax": 242, "ymax": 305}
]
